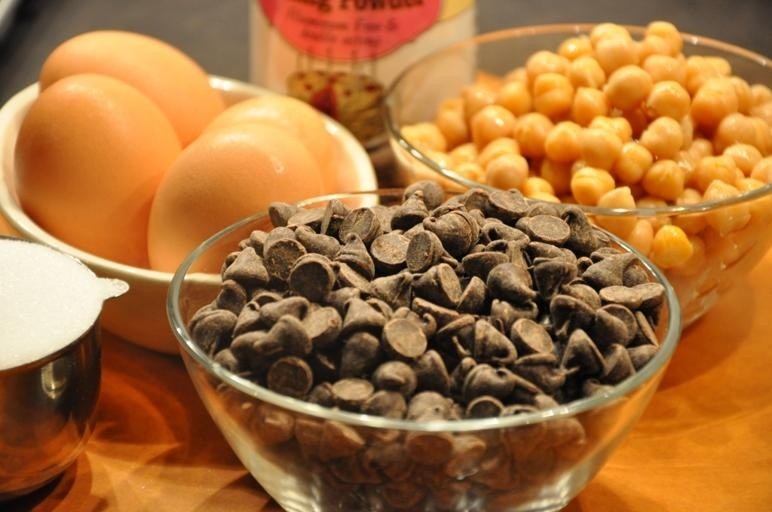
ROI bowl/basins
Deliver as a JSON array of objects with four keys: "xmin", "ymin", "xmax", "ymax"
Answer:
[
  {"xmin": 0, "ymin": 72, "xmax": 380, "ymax": 355},
  {"xmin": 163, "ymin": 187, "xmax": 683, "ymax": 511}
]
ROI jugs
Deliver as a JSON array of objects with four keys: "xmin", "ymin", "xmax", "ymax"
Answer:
[{"xmin": 0, "ymin": 238, "xmax": 129, "ymax": 507}]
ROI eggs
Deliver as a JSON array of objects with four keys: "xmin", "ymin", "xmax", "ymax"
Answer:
[{"xmin": 12, "ymin": 26, "xmax": 361, "ymax": 275}]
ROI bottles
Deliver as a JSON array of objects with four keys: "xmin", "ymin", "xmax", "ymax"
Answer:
[{"xmin": 248, "ymin": 0, "xmax": 479, "ymax": 184}]
